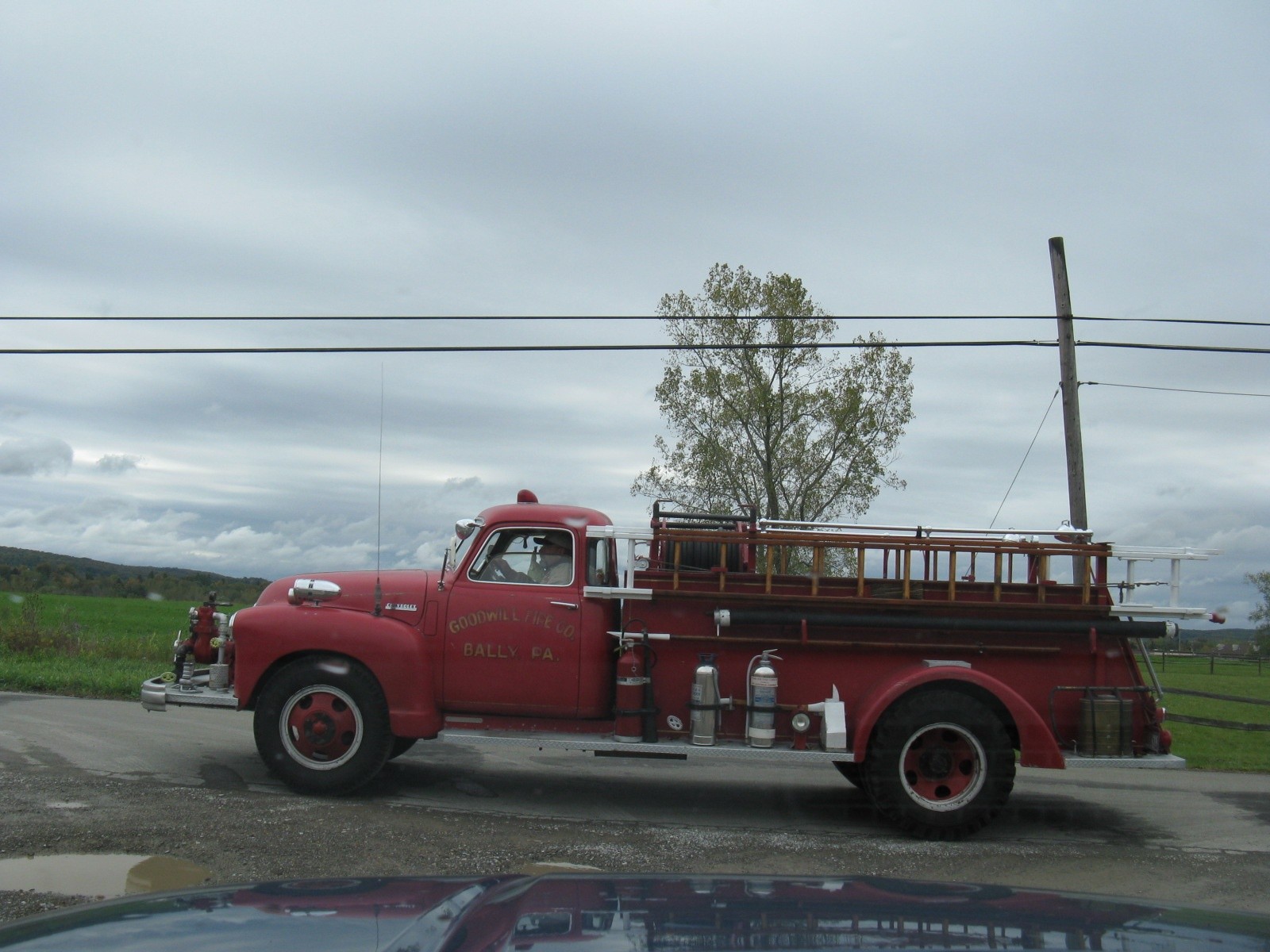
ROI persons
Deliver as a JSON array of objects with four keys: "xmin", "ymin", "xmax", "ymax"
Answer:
[{"xmin": 493, "ymin": 531, "xmax": 572, "ymax": 585}]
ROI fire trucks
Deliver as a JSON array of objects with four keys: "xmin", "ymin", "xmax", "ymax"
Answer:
[{"xmin": 229, "ymin": 490, "xmax": 1225, "ymax": 840}]
